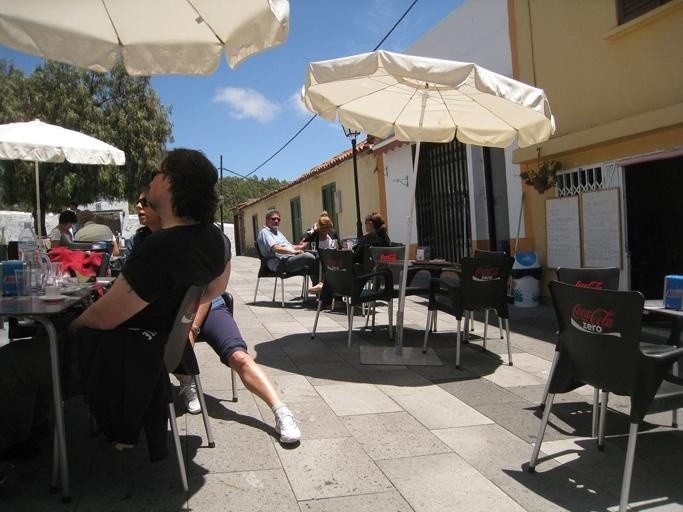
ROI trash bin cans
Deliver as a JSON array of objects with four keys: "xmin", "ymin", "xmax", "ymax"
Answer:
[{"xmin": 512, "ymin": 252, "xmax": 542, "ymax": 308}]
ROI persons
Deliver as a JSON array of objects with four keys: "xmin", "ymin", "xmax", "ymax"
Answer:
[
  {"xmin": 307, "ymin": 212, "xmax": 390, "ymax": 294},
  {"xmin": 69, "ymin": 202, "xmax": 80, "ymax": 215},
  {"xmin": 299, "ymin": 210, "xmax": 343, "ymax": 305},
  {"xmin": 48, "ymin": 210, "xmax": 76, "ymax": 248},
  {"xmin": 0, "ymin": 148, "xmax": 231, "ymax": 461},
  {"xmin": 255, "ymin": 210, "xmax": 345, "ymax": 309},
  {"xmin": 124, "ymin": 190, "xmax": 301, "ymax": 444},
  {"xmin": 74, "ymin": 210, "xmax": 119, "ymax": 257}
]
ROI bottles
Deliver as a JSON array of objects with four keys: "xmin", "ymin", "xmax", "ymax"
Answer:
[
  {"xmin": 117, "ymin": 233, "xmax": 125, "ymax": 248},
  {"xmin": 18, "ymin": 221, "xmax": 38, "ymax": 260}
]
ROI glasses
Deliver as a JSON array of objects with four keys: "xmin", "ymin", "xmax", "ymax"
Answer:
[
  {"xmin": 268, "ymin": 217, "xmax": 281, "ymax": 222},
  {"xmin": 136, "ymin": 197, "xmax": 152, "ymax": 207}
]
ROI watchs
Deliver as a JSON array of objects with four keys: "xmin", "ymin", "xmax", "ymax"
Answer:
[{"xmin": 190, "ymin": 325, "xmax": 200, "ymax": 336}]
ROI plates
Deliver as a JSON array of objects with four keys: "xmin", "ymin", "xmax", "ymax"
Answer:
[
  {"xmin": 38, "ymin": 296, "xmax": 68, "ymax": 302},
  {"xmin": 62, "ymin": 285, "xmax": 83, "ymax": 294}
]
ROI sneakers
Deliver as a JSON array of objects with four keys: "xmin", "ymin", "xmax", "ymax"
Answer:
[
  {"xmin": 274, "ymin": 408, "xmax": 301, "ymax": 444},
  {"xmin": 179, "ymin": 374, "xmax": 201, "ymax": 415},
  {"xmin": 308, "ymin": 283, "xmax": 323, "ymax": 294},
  {"xmin": 334, "ymin": 300, "xmax": 346, "ymax": 309}
]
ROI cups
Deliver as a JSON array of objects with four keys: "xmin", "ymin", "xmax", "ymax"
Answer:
[
  {"xmin": 12, "ymin": 260, "xmax": 64, "ymax": 299},
  {"xmin": 310, "ymin": 239, "xmax": 353, "ymax": 251},
  {"xmin": 311, "ymin": 242, "xmax": 315, "ymax": 250},
  {"xmin": 44, "ymin": 286, "xmax": 61, "ymax": 297}
]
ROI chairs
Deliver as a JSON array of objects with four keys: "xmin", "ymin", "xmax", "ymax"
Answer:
[
  {"xmin": 527, "ymin": 267, "xmax": 683, "ymax": 511},
  {"xmin": 253, "ymin": 237, "xmax": 515, "ymax": 369}
]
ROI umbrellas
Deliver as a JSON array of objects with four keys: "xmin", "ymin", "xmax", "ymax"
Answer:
[
  {"xmin": 0, "ymin": 118, "xmax": 125, "ymax": 253},
  {"xmin": 300, "ymin": 48, "xmax": 555, "ymax": 366},
  {"xmin": 0, "ymin": 0, "xmax": 289, "ymax": 77}
]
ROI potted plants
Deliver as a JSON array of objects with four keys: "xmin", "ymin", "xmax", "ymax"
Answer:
[{"xmin": 519, "ymin": 147, "xmax": 562, "ymax": 193}]
